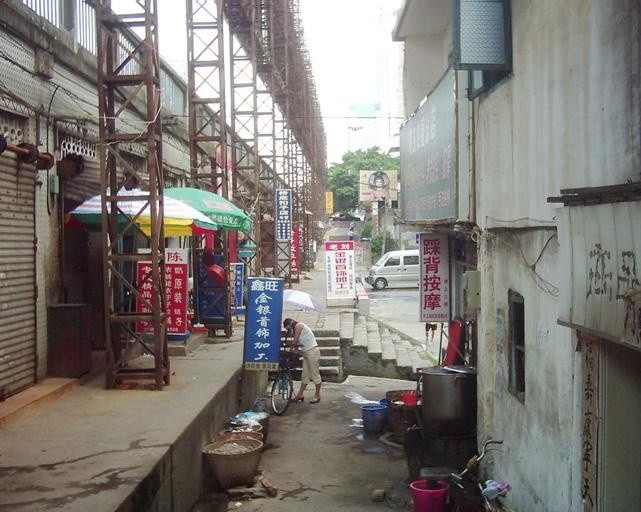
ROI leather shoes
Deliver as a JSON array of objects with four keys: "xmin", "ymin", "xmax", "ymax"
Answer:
[{"xmin": 292, "ymin": 394, "xmax": 321, "ymax": 404}]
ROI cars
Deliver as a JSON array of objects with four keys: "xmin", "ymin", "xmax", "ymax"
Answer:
[{"xmin": 329, "ymin": 213, "xmax": 361, "ymax": 222}]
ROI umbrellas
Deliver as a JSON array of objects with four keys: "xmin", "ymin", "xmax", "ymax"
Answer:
[
  {"xmin": 164, "ymin": 186, "xmax": 254, "ymax": 231},
  {"xmin": 69, "ymin": 186, "xmax": 220, "ymax": 365},
  {"xmin": 283, "ymin": 286, "xmax": 326, "ymax": 329}
]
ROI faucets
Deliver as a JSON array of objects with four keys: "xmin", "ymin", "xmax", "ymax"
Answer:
[{"xmin": 406, "ymin": 424, "xmax": 423, "ymax": 433}]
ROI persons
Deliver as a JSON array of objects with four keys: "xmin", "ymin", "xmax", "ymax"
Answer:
[
  {"xmin": 283, "ymin": 317, "xmax": 324, "ymax": 402},
  {"xmin": 348, "ymin": 228, "xmax": 355, "ymax": 241},
  {"xmin": 199, "ymin": 252, "xmax": 236, "ymax": 334},
  {"xmin": 350, "ymin": 219, "xmax": 356, "ymax": 232},
  {"xmin": 368, "ymin": 171, "xmax": 392, "ymax": 201}
]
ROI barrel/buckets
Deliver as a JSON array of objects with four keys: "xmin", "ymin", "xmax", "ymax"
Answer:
[
  {"xmin": 391, "ymin": 399, "xmax": 424, "ymax": 443},
  {"xmin": 379, "ymin": 398, "xmax": 391, "ymax": 425},
  {"xmin": 386, "ymin": 389, "xmax": 424, "ymax": 430},
  {"xmin": 360, "ymin": 403, "xmax": 387, "ymax": 432},
  {"xmin": 410, "ymin": 481, "xmax": 450, "ymax": 512}
]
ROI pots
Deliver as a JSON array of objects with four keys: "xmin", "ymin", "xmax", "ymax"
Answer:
[{"xmin": 421, "ymin": 363, "xmax": 477, "ymax": 438}]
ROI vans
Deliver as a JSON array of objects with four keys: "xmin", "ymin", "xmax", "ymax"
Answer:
[{"xmin": 365, "ymin": 249, "xmax": 421, "ymax": 290}]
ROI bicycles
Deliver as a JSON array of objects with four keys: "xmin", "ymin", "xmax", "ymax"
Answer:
[
  {"xmin": 270, "ymin": 343, "xmax": 305, "ymax": 416},
  {"xmin": 451, "ymin": 438, "xmax": 513, "ymax": 511}
]
